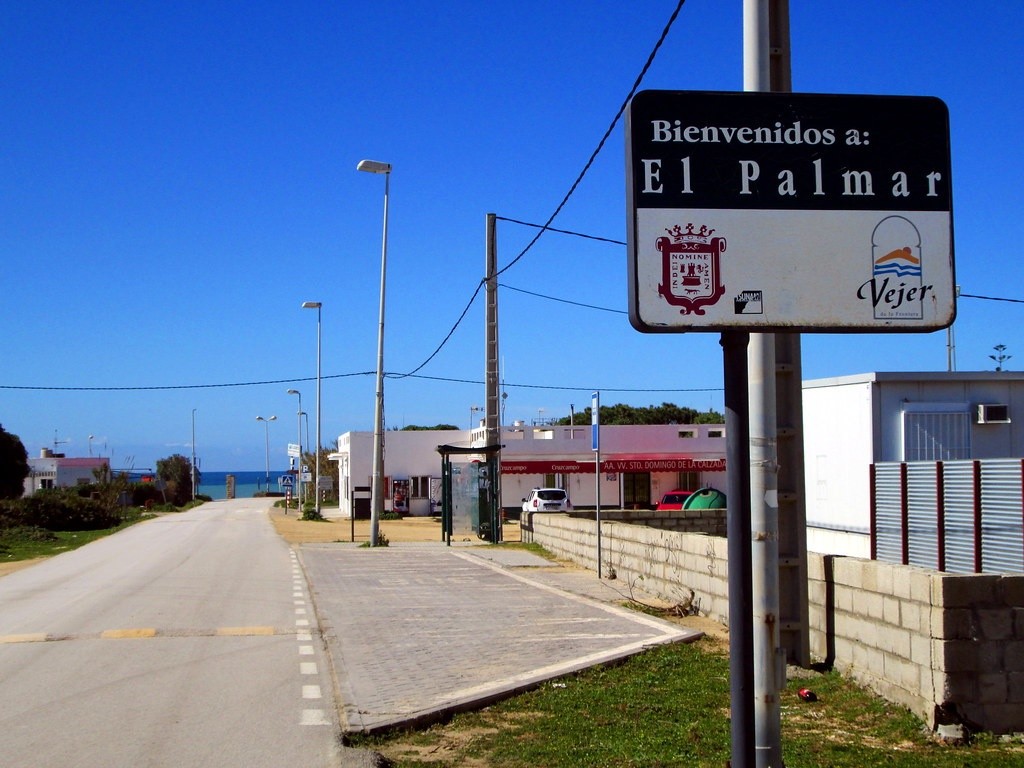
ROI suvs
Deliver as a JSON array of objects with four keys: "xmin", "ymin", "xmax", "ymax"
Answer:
[{"xmin": 521, "ymin": 487, "xmax": 575, "ymax": 513}]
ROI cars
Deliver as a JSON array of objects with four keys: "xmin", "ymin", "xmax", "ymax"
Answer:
[{"xmin": 656, "ymin": 489, "xmax": 696, "ymax": 511}]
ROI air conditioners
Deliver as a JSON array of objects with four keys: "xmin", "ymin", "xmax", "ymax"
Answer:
[{"xmin": 974, "ymin": 402, "xmax": 1011, "ymax": 424}]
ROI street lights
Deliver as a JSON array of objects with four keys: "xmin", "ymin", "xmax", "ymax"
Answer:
[
  {"xmin": 301, "ymin": 301, "xmax": 323, "ymax": 515},
  {"xmin": 88, "ymin": 436, "xmax": 94, "ymax": 458},
  {"xmin": 357, "ymin": 159, "xmax": 393, "ymax": 550},
  {"xmin": 191, "ymin": 408, "xmax": 197, "ymax": 501},
  {"xmin": 287, "ymin": 388, "xmax": 301, "ymax": 511},
  {"xmin": 297, "ymin": 411, "xmax": 309, "ymax": 454},
  {"xmin": 256, "ymin": 415, "xmax": 277, "ymax": 492}
]
